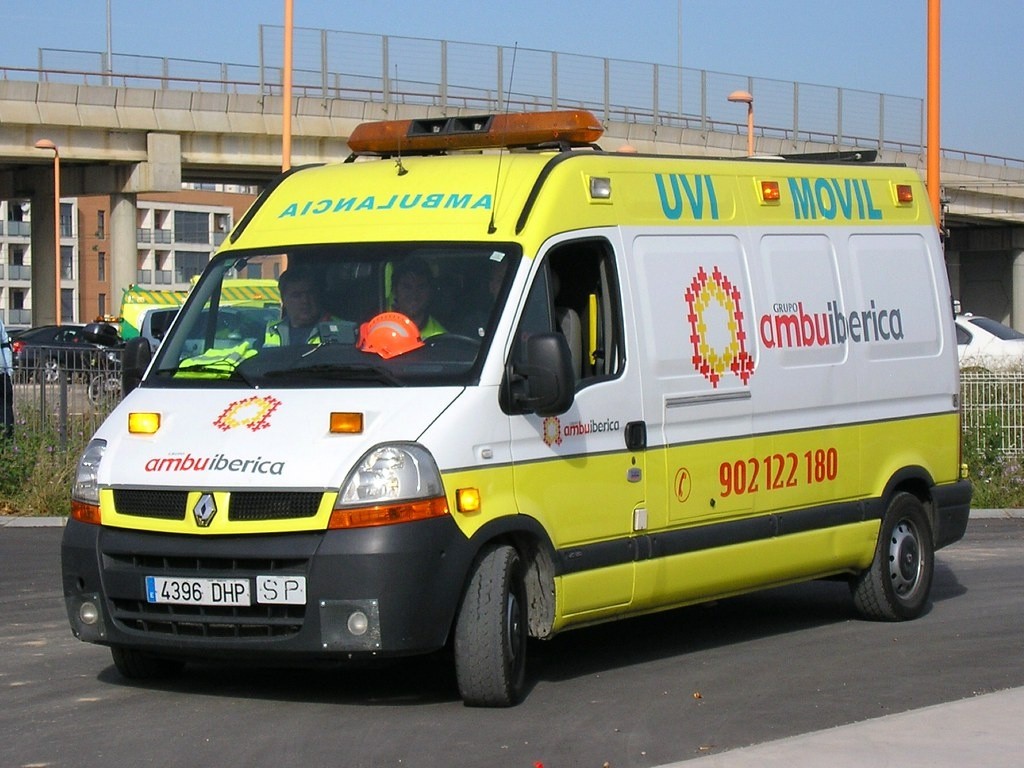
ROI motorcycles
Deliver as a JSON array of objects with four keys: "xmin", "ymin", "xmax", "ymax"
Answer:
[{"xmin": 86, "ymin": 343, "xmax": 122, "ymax": 406}]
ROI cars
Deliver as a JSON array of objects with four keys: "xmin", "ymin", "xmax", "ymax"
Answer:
[
  {"xmin": 10, "ymin": 324, "xmax": 102, "ymax": 385},
  {"xmin": 955, "ymin": 312, "xmax": 1024, "ymax": 402}
]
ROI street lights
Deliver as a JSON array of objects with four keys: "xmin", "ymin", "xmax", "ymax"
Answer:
[
  {"xmin": 34, "ymin": 138, "xmax": 61, "ymax": 328},
  {"xmin": 726, "ymin": 89, "xmax": 754, "ymax": 158}
]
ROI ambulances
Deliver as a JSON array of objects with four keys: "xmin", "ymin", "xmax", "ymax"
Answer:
[
  {"xmin": 117, "ymin": 276, "xmax": 290, "ymax": 365},
  {"xmin": 59, "ymin": 110, "xmax": 972, "ymax": 706}
]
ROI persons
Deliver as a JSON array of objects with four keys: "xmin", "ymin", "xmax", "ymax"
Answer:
[
  {"xmin": 253, "ymin": 265, "xmax": 344, "ymax": 346},
  {"xmin": 0, "ymin": 320, "xmax": 20, "ymax": 442},
  {"xmin": 390, "ymin": 262, "xmax": 448, "ymax": 339}
]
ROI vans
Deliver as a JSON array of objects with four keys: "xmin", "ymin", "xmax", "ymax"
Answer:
[{"xmin": 140, "ymin": 304, "xmax": 280, "ymax": 362}]
